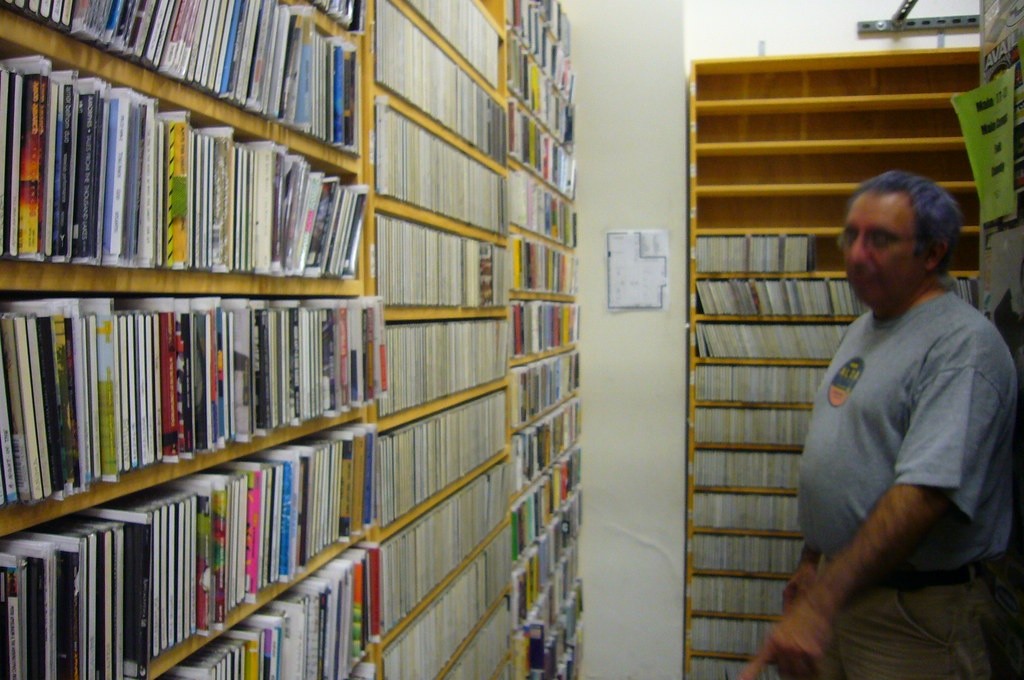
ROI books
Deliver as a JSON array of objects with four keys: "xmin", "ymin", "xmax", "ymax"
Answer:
[
  {"xmin": 0, "ymin": 0, "xmax": 587, "ymax": 679},
  {"xmin": 687, "ymin": 235, "xmax": 983, "ymax": 680}
]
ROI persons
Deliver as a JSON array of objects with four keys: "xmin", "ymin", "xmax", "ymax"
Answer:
[{"xmin": 740, "ymin": 169, "xmax": 1015, "ymax": 680}]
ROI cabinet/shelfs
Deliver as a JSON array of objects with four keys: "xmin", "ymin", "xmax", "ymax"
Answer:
[
  {"xmin": 0, "ymin": 0, "xmax": 578, "ymax": 680},
  {"xmin": 685, "ymin": 49, "xmax": 983, "ymax": 680}
]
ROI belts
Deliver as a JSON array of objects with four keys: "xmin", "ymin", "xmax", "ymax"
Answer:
[{"xmin": 804, "ymin": 543, "xmax": 983, "ymax": 589}]
